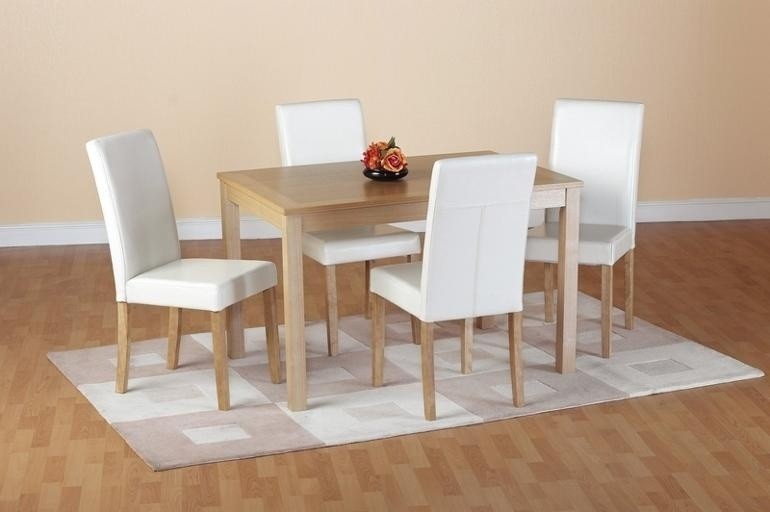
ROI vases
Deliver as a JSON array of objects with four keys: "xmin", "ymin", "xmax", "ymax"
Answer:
[{"xmin": 363, "ymin": 167, "xmax": 409, "ymax": 181}]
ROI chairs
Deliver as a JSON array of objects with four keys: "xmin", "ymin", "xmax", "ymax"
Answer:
[
  {"xmin": 275, "ymin": 98, "xmax": 421, "ymax": 355},
  {"xmin": 85, "ymin": 128, "xmax": 280, "ymax": 411},
  {"xmin": 369, "ymin": 152, "xmax": 538, "ymax": 421},
  {"xmin": 526, "ymin": 99, "xmax": 645, "ymax": 359}
]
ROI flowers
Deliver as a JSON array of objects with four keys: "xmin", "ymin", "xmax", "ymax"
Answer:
[{"xmin": 360, "ymin": 135, "xmax": 408, "ymax": 173}]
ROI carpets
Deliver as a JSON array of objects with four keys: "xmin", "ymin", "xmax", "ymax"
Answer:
[{"xmin": 44, "ymin": 286, "xmax": 765, "ymax": 473}]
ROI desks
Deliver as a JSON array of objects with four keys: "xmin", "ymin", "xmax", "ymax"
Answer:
[{"xmin": 217, "ymin": 152, "xmax": 585, "ymax": 412}]
